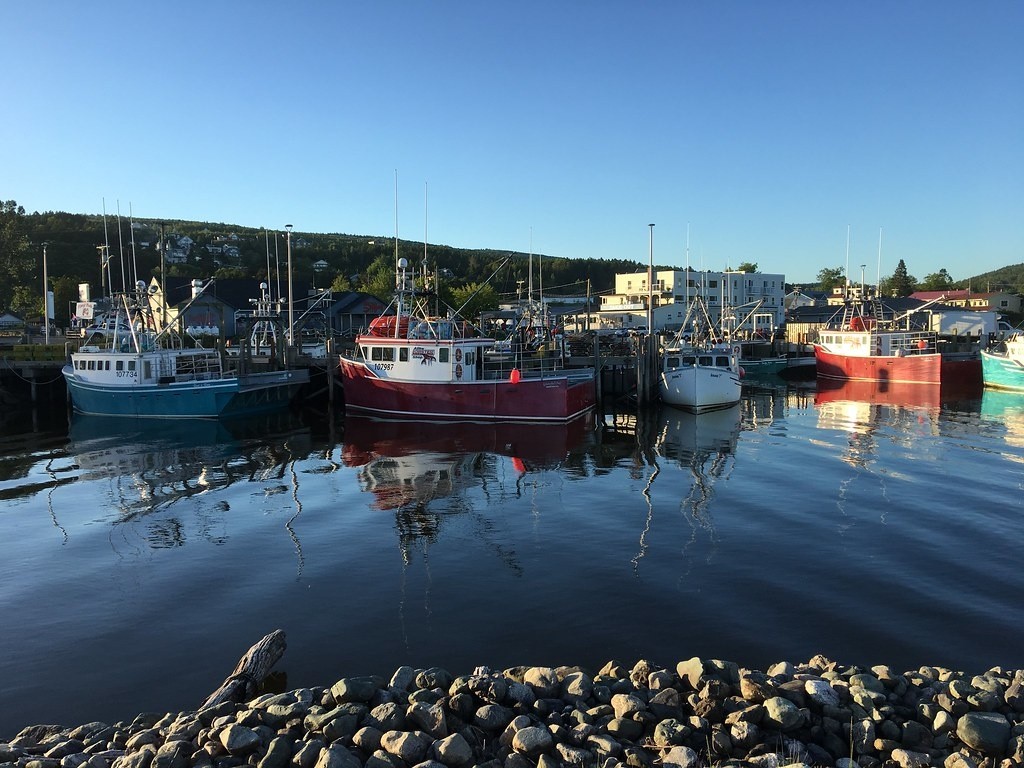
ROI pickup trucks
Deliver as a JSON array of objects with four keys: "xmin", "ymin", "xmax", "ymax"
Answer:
[{"xmin": 80, "ymin": 322, "xmax": 135, "ymax": 340}]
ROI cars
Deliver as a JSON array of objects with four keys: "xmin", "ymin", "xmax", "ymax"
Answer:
[
  {"xmin": 996, "ymin": 321, "xmax": 1023, "ymax": 341},
  {"xmin": 615, "ymin": 325, "xmax": 649, "ymax": 336}
]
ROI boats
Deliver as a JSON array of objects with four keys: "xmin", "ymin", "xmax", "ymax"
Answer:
[
  {"xmin": 813, "ymin": 376, "xmax": 982, "ymax": 471},
  {"xmin": 70, "ymin": 411, "xmax": 314, "ymax": 582},
  {"xmin": 339, "ymin": 167, "xmax": 600, "ymax": 422},
  {"xmin": 980, "ymin": 386, "xmax": 1023, "ymax": 428},
  {"xmin": 980, "ymin": 327, "xmax": 1024, "ymax": 390},
  {"xmin": 340, "ymin": 408, "xmax": 599, "ymax": 644},
  {"xmin": 812, "ymin": 225, "xmax": 980, "ymax": 384},
  {"xmin": 659, "ymin": 276, "xmax": 744, "ymax": 413},
  {"xmin": 656, "ymin": 403, "xmax": 742, "ymax": 565},
  {"xmin": 60, "ymin": 198, "xmax": 312, "ymax": 418}
]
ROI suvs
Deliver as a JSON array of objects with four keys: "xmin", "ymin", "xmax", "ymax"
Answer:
[{"xmin": 674, "ymin": 327, "xmax": 694, "ymax": 341}]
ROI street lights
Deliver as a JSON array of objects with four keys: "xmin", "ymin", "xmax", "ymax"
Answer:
[
  {"xmin": 861, "ymin": 265, "xmax": 866, "ymax": 295},
  {"xmin": 285, "ymin": 225, "xmax": 295, "ymax": 346},
  {"xmin": 648, "ymin": 223, "xmax": 655, "ymax": 335},
  {"xmin": 39, "ymin": 242, "xmax": 49, "ymax": 343}
]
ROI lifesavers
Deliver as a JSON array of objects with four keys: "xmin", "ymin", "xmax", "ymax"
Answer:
[
  {"xmin": 456, "ymin": 348, "xmax": 462, "ymax": 361},
  {"xmin": 456, "ymin": 364, "xmax": 462, "ymax": 378}
]
